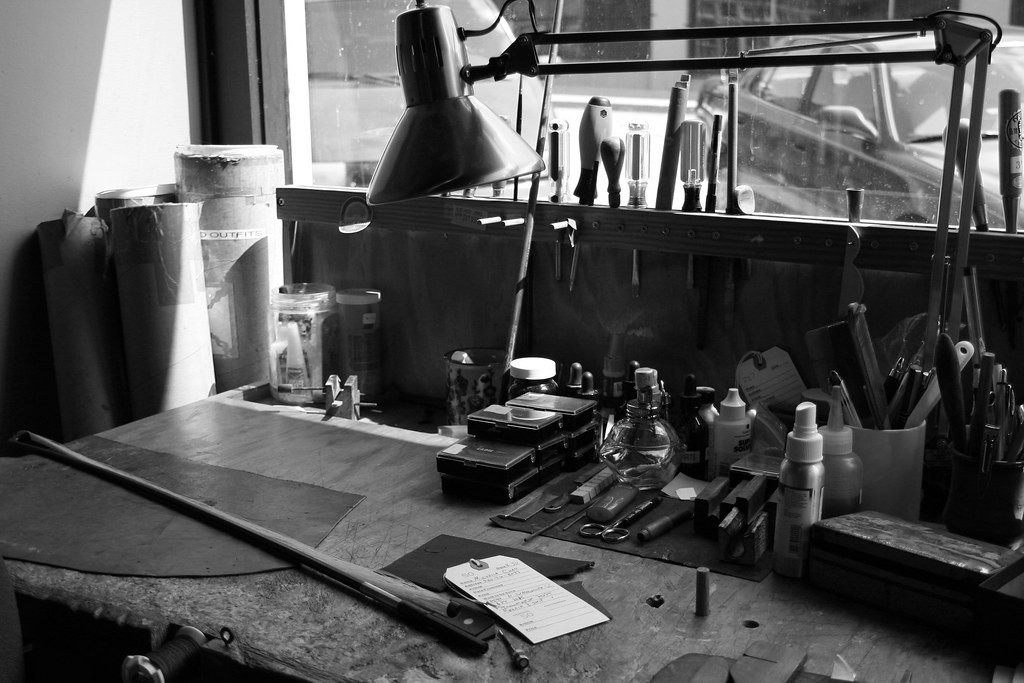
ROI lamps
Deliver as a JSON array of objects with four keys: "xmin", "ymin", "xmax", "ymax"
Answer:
[{"xmin": 367, "ymin": 0, "xmax": 1023, "ymax": 470}]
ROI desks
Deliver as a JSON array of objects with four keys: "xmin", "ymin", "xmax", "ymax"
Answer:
[{"xmin": 0, "ymin": 377, "xmax": 1024, "ymax": 683}]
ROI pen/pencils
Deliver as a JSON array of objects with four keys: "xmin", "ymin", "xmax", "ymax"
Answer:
[{"xmin": 830, "ymin": 251, "xmax": 1024, "ymax": 473}]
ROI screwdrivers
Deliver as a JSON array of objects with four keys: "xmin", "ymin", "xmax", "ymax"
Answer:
[
  {"xmin": 679, "ymin": 118, "xmax": 707, "ymax": 292},
  {"xmin": 568, "ymin": 96, "xmax": 648, "ymax": 300},
  {"xmin": 943, "ymin": 120, "xmax": 1010, "ymax": 331},
  {"xmin": 548, "ymin": 122, "xmax": 564, "ymax": 283}
]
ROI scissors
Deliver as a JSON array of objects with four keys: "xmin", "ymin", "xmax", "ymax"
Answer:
[{"xmin": 579, "ymin": 498, "xmax": 655, "ymax": 545}]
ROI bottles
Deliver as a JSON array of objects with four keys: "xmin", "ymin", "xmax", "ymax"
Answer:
[{"xmin": 845, "ymin": 422, "xmax": 928, "ymax": 524}]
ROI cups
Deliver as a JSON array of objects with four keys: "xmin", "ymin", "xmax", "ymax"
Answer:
[
  {"xmin": 336, "ymin": 288, "xmax": 382, "ymax": 405},
  {"xmin": 441, "ymin": 347, "xmax": 508, "ymax": 433},
  {"xmin": 269, "ymin": 283, "xmax": 337, "ymax": 408}
]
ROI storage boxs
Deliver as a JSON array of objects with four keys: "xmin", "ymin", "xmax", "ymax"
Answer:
[
  {"xmin": 437, "ymin": 392, "xmax": 599, "ymax": 507},
  {"xmin": 804, "ymin": 511, "xmax": 1024, "ymax": 638},
  {"xmin": 978, "ymin": 556, "xmax": 1023, "ymax": 657}
]
